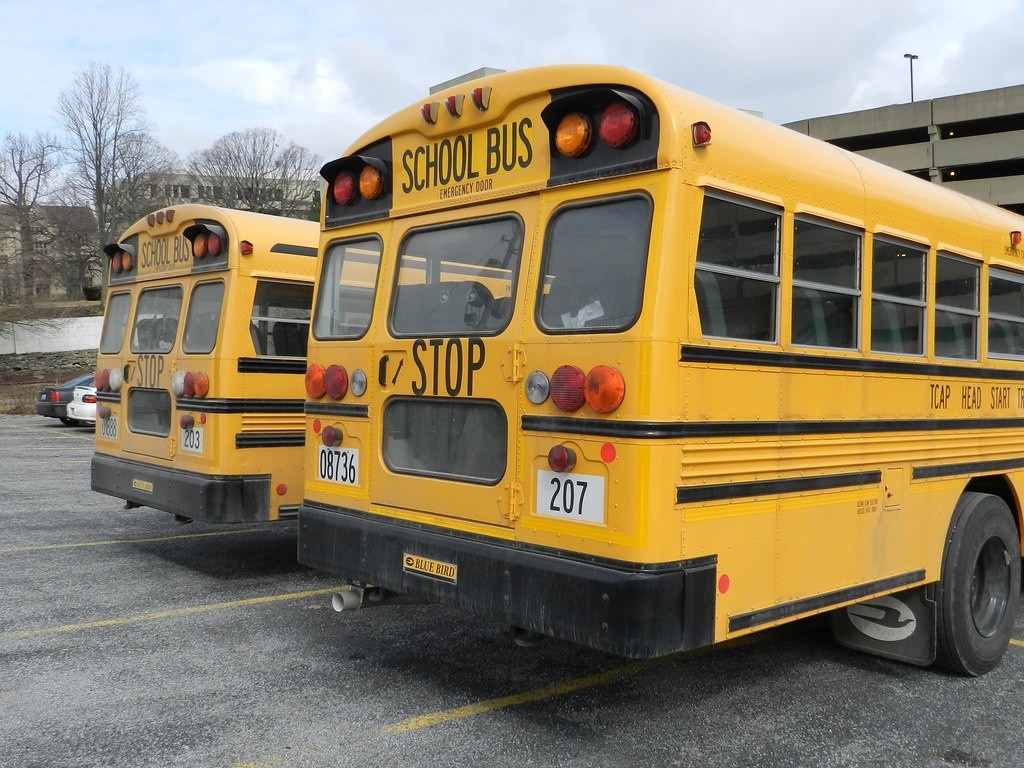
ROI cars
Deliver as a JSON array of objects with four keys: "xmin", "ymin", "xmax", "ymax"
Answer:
[
  {"xmin": 34, "ymin": 372, "xmax": 95, "ymax": 426},
  {"xmin": 65, "ymin": 385, "xmax": 97, "ymax": 425}
]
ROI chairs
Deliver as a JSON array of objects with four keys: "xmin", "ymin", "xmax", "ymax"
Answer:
[
  {"xmin": 133, "ymin": 316, "xmax": 311, "ymax": 360},
  {"xmin": 390, "ymin": 267, "xmax": 1019, "ymax": 359}
]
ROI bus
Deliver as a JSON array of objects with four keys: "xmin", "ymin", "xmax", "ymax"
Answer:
[
  {"xmin": 298, "ymin": 63, "xmax": 1024, "ymax": 678},
  {"xmin": 87, "ymin": 202, "xmax": 559, "ymax": 526}
]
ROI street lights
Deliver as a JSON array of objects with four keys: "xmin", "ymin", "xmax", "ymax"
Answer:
[{"xmin": 904, "ymin": 54, "xmax": 919, "ymax": 102}]
